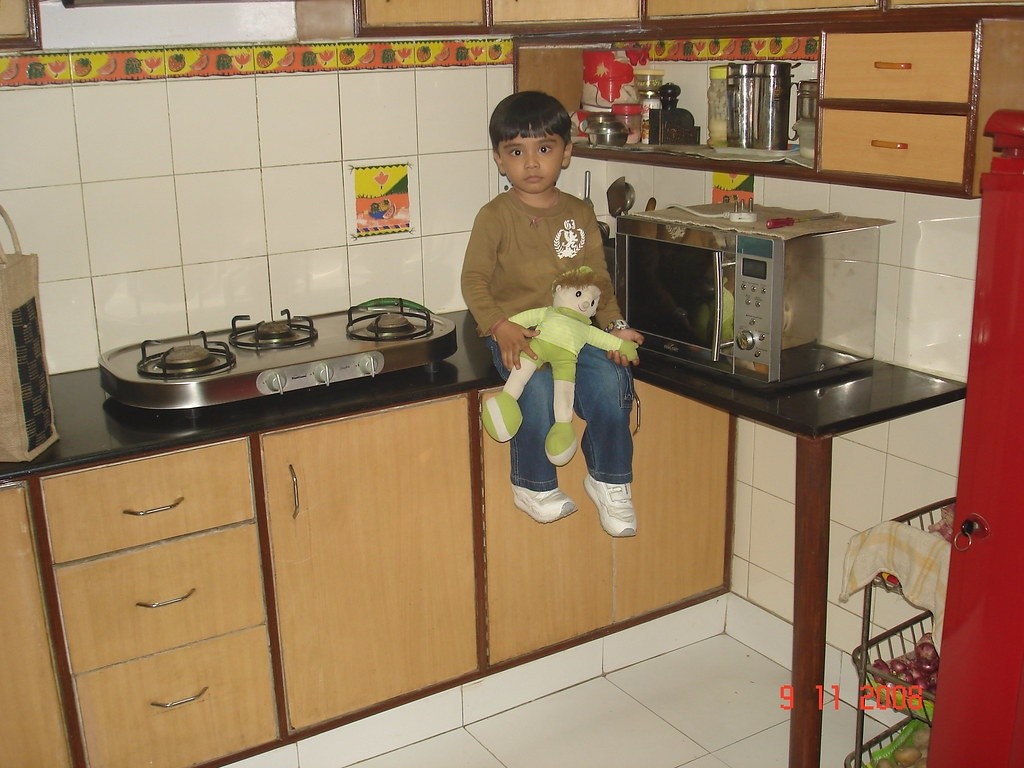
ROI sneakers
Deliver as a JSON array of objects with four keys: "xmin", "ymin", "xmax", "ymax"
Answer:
[
  {"xmin": 583, "ymin": 474, "xmax": 636, "ymax": 537},
  {"xmin": 512, "ymin": 485, "xmax": 578, "ymax": 523}
]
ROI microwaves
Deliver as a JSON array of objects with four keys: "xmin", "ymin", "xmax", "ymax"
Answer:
[{"xmin": 616, "ymin": 199, "xmax": 881, "ymax": 384}]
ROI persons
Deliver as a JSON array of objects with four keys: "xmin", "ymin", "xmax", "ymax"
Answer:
[{"xmin": 462, "ymin": 91, "xmax": 644, "ymax": 536}]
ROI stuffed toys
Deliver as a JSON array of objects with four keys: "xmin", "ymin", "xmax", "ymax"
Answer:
[{"xmin": 480, "ymin": 266, "xmax": 638, "ymax": 466}]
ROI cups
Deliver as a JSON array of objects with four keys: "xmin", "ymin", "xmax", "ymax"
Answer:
[{"xmin": 788, "ymin": 79, "xmax": 818, "ymax": 142}]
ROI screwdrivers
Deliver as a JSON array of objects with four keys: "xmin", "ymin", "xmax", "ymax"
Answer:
[{"xmin": 766, "ymin": 213, "xmax": 838, "ymax": 228}]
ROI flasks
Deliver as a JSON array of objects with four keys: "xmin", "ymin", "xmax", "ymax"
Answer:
[
  {"xmin": 752, "ymin": 61, "xmax": 791, "ymax": 149},
  {"xmin": 726, "ymin": 63, "xmax": 756, "ymax": 149}
]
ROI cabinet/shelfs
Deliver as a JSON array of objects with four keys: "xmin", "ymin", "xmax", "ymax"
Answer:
[
  {"xmin": 0, "ymin": 0, "xmax": 42, "ymax": 49},
  {"xmin": 844, "ymin": 497, "xmax": 957, "ymax": 768},
  {"xmin": 0, "ymin": 307, "xmax": 737, "ymax": 768},
  {"xmin": 295, "ymin": 0, "xmax": 1024, "ymax": 201}
]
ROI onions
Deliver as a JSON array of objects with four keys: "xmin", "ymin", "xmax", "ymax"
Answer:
[{"xmin": 870, "ymin": 631, "xmax": 938, "ymax": 697}]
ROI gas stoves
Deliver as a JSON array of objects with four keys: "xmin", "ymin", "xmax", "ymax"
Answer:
[{"xmin": 98, "ymin": 298, "xmax": 457, "ymax": 410}]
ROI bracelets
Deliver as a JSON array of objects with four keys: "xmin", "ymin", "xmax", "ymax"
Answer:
[
  {"xmin": 491, "ymin": 318, "xmax": 507, "ymax": 341},
  {"xmin": 604, "ymin": 320, "xmax": 630, "ymax": 333}
]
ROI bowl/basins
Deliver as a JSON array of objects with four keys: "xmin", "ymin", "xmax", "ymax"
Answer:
[{"xmin": 584, "ymin": 122, "xmax": 629, "ymax": 146}]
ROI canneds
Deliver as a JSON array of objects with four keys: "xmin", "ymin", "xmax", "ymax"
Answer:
[{"xmin": 640, "ymin": 98, "xmax": 662, "ymax": 144}]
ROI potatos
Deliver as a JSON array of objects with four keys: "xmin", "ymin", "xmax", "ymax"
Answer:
[{"xmin": 876, "ymin": 726, "xmax": 930, "ymax": 768}]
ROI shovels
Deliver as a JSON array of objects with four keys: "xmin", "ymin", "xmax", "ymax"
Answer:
[{"xmin": 605, "ymin": 176, "xmax": 626, "ymax": 220}]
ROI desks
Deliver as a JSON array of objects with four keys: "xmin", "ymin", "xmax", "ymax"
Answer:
[{"xmin": 632, "ymin": 352, "xmax": 969, "ymax": 768}]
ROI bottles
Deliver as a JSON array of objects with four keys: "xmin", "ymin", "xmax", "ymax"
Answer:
[
  {"xmin": 792, "ymin": 118, "xmax": 815, "ymax": 160},
  {"xmin": 633, "ymin": 69, "xmax": 665, "ymax": 90},
  {"xmin": 587, "ymin": 112, "xmax": 613, "ymax": 123},
  {"xmin": 611, "ymin": 91, "xmax": 666, "ymax": 143},
  {"xmin": 706, "ymin": 65, "xmax": 731, "ymax": 146}
]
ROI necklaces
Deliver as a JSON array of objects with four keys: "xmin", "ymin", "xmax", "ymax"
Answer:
[{"xmin": 513, "ymin": 189, "xmax": 557, "ymax": 224}]
